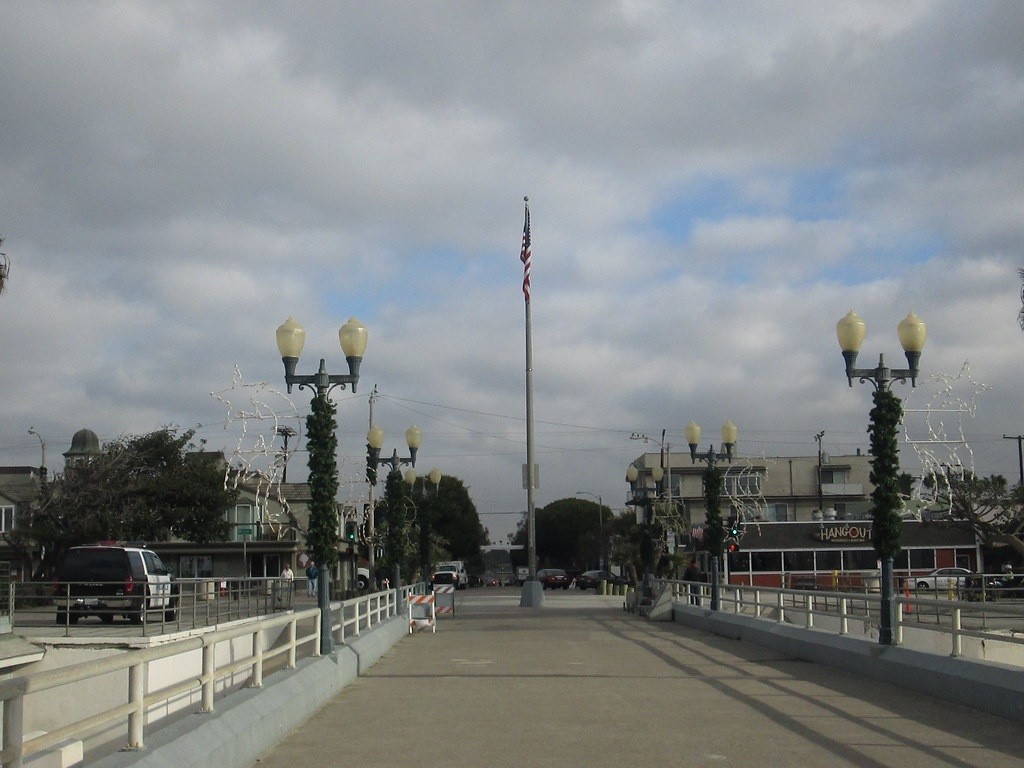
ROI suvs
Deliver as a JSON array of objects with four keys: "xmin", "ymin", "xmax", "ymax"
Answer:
[{"xmin": 56, "ymin": 540, "xmax": 179, "ymax": 625}]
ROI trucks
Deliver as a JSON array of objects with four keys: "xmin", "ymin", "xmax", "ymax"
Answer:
[{"xmin": 429, "ymin": 559, "xmax": 468, "ymax": 591}]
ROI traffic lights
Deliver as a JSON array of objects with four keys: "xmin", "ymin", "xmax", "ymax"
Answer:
[
  {"xmin": 728, "ymin": 516, "xmax": 740, "ymax": 536},
  {"xmin": 728, "ymin": 540, "xmax": 738, "ymax": 553},
  {"xmin": 345, "ymin": 521, "xmax": 357, "ymax": 541}
]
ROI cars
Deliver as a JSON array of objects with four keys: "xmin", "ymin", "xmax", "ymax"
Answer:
[
  {"xmin": 467, "ymin": 573, "xmax": 523, "ymax": 586},
  {"xmin": 903, "ymin": 567, "xmax": 978, "ymax": 593},
  {"xmin": 576, "ymin": 569, "xmax": 631, "ymax": 590},
  {"xmin": 537, "ymin": 568, "xmax": 571, "ymax": 590}
]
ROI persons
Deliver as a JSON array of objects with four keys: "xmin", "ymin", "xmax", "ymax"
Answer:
[
  {"xmin": 683, "ymin": 560, "xmax": 703, "ymax": 606},
  {"xmin": 1006, "ymin": 568, "xmax": 1016, "ymax": 598},
  {"xmin": 281, "ymin": 564, "xmax": 294, "ymax": 599},
  {"xmin": 306, "ymin": 562, "xmax": 318, "ymax": 596},
  {"xmin": 965, "ymin": 572, "xmax": 978, "ymax": 601}
]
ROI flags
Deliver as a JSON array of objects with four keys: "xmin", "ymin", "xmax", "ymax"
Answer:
[{"xmin": 520, "ymin": 211, "xmax": 531, "ymax": 301}]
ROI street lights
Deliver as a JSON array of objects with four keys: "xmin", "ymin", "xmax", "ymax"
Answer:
[
  {"xmin": 403, "ymin": 463, "xmax": 441, "ymax": 595},
  {"xmin": 627, "ymin": 461, "xmax": 665, "ymax": 600},
  {"xmin": 833, "ymin": 310, "xmax": 929, "ymax": 646},
  {"xmin": 629, "ymin": 432, "xmax": 672, "ymax": 509},
  {"xmin": 684, "ymin": 417, "xmax": 739, "ymax": 609},
  {"xmin": 370, "ymin": 421, "xmax": 421, "ymax": 617},
  {"xmin": 29, "ymin": 429, "xmax": 44, "ymax": 467},
  {"xmin": 576, "ymin": 491, "xmax": 605, "ymax": 569},
  {"xmin": 275, "ymin": 315, "xmax": 370, "ymax": 654}
]
